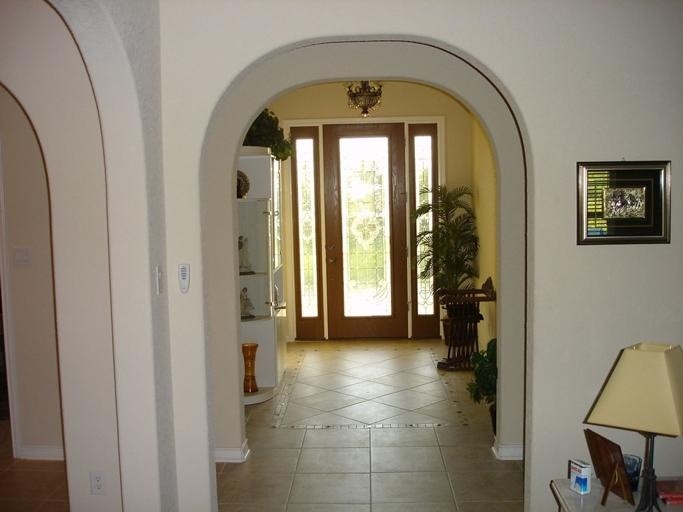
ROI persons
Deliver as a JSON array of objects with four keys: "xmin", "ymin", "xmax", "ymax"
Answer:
[
  {"xmin": 240, "ymin": 287, "xmax": 254, "ymax": 317},
  {"xmin": 238, "ymin": 235, "xmax": 251, "ymax": 267}
]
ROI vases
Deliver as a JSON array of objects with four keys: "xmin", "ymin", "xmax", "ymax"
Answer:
[{"xmin": 241, "ymin": 342, "xmax": 260, "ymax": 394}]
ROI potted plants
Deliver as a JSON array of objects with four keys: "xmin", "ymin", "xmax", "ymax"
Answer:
[
  {"xmin": 411, "ymin": 182, "xmax": 481, "ymax": 348},
  {"xmin": 463, "ymin": 338, "xmax": 496, "ymax": 436}
]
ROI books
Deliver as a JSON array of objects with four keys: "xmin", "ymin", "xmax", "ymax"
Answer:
[{"xmin": 657, "ymin": 480, "xmax": 683, "ymax": 504}]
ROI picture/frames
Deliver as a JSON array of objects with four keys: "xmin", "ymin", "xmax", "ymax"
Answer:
[{"xmin": 572, "ymin": 159, "xmax": 672, "ymax": 246}]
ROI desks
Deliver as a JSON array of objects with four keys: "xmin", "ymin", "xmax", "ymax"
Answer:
[{"xmin": 547, "ymin": 475, "xmax": 682, "ymax": 512}]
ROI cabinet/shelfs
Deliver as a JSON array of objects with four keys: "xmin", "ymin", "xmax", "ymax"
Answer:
[
  {"xmin": 231, "ymin": 147, "xmax": 273, "ymax": 408},
  {"xmin": 271, "ymin": 152, "xmax": 290, "ymax": 392}
]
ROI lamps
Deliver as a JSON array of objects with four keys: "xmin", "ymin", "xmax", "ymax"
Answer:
[
  {"xmin": 582, "ymin": 339, "xmax": 682, "ymax": 512},
  {"xmin": 342, "ymin": 78, "xmax": 388, "ymax": 118}
]
